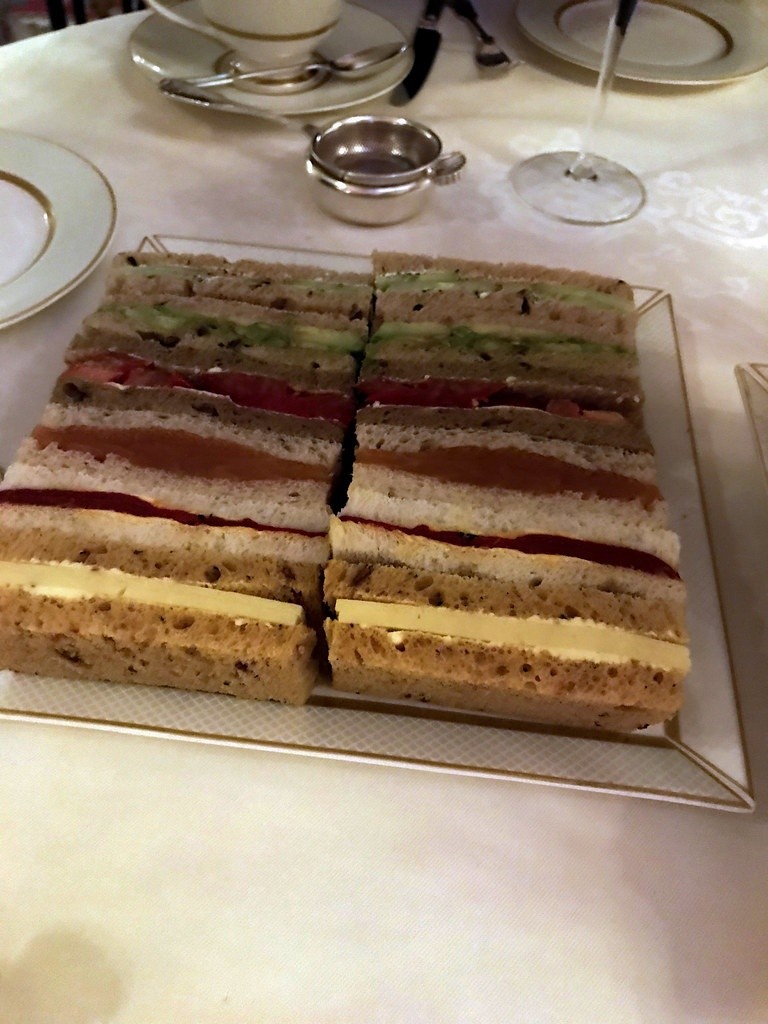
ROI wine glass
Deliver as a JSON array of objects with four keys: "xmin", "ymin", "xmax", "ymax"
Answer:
[{"xmin": 507, "ymin": 0, "xmax": 649, "ymax": 225}]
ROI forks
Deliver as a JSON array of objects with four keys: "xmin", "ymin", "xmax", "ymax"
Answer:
[{"xmin": 444, "ymin": 0, "xmax": 524, "ymax": 71}]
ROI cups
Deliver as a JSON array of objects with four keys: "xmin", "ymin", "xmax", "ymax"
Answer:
[{"xmin": 144, "ymin": 0, "xmax": 344, "ymax": 65}]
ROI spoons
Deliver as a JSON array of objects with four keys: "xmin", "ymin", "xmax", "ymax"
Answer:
[{"xmin": 157, "ymin": 41, "xmax": 409, "ymax": 97}]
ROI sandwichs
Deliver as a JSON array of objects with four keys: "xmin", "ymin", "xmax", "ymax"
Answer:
[{"xmin": 0, "ymin": 250, "xmax": 691, "ymax": 736}]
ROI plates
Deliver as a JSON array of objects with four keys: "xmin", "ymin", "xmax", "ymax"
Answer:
[
  {"xmin": 0, "ymin": 127, "xmax": 119, "ymax": 330},
  {"xmin": 0, "ymin": 231, "xmax": 755, "ymax": 816},
  {"xmin": 511, "ymin": 0, "xmax": 768, "ymax": 86},
  {"xmin": 127, "ymin": 0, "xmax": 414, "ymax": 115}
]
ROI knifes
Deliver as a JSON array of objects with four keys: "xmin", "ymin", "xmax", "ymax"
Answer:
[{"xmin": 401, "ymin": 0, "xmax": 446, "ymax": 107}]
